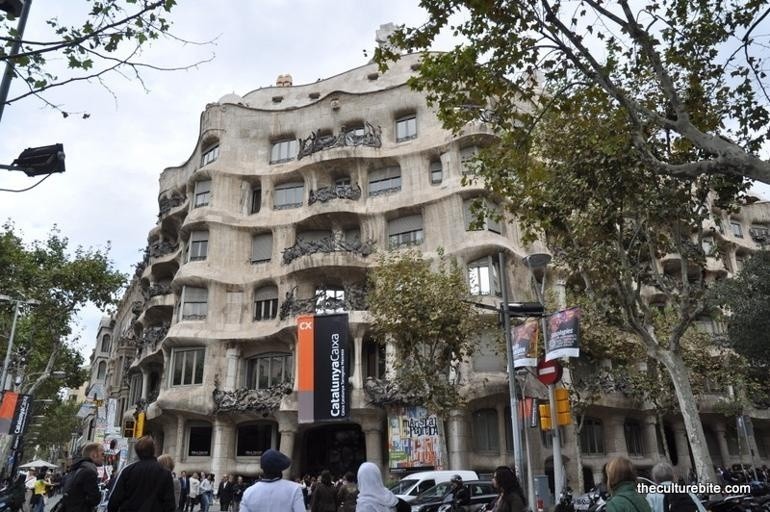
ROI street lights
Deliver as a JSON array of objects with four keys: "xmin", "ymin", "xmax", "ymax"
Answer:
[
  {"xmin": 522, "ymin": 237, "xmax": 567, "ymax": 507},
  {"xmin": 14, "ymin": 375, "xmax": 21, "ymax": 394},
  {"xmin": 1, "ymin": 293, "xmax": 43, "ymax": 402},
  {"xmin": 495, "ymin": 249, "xmax": 544, "ymax": 497}
]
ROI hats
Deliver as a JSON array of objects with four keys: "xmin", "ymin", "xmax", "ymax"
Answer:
[{"xmin": 259, "ymin": 448, "xmax": 291, "ymax": 473}]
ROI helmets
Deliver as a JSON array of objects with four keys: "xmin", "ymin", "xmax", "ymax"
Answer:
[{"xmin": 450, "ymin": 474, "xmax": 463, "ymax": 489}]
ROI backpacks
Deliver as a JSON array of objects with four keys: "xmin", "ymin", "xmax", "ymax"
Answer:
[{"xmin": 651, "ymin": 486, "xmax": 700, "ymax": 512}]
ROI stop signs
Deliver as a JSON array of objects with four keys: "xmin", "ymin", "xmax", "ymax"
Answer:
[{"xmin": 537, "ymin": 358, "xmax": 563, "ymax": 384}]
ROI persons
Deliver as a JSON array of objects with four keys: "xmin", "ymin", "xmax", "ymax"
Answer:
[{"xmin": 1, "ymin": 435, "xmax": 706, "ymax": 512}]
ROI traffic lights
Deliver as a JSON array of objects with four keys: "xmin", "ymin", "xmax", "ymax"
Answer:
[{"xmin": 123, "ymin": 417, "xmax": 136, "ymax": 439}]
